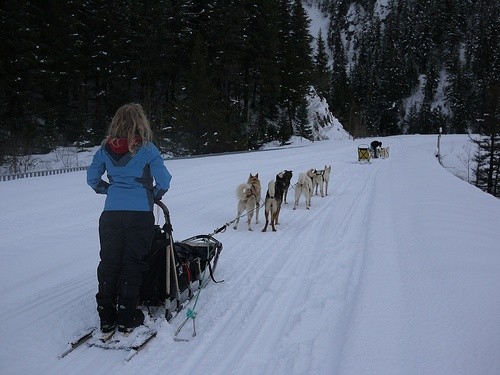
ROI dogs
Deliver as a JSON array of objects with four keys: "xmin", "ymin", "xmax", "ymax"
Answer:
[
  {"xmin": 261, "ymin": 174, "xmax": 285, "ymax": 232},
  {"xmin": 294, "ymin": 169, "xmax": 317, "ymax": 210},
  {"xmin": 276, "ymin": 169, "xmax": 293, "ymax": 204},
  {"xmin": 233, "ymin": 173, "xmax": 261, "ymax": 231},
  {"xmin": 312, "ymin": 165, "xmax": 331, "ymax": 197},
  {"xmin": 370, "ymin": 146, "xmax": 390, "ymax": 159}
]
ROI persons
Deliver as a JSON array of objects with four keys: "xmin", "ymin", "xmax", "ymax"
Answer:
[
  {"xmin": 371, "ymin": 141, "xmax": 382, "ymax": 158},
  {"xmin": 87, "ymin": 104, "xmax": 172, "ymax": 333}
]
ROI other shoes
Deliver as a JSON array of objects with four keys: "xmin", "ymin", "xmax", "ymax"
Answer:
[
  {"xmin": 374, "ymin": 156, "xmax": 378, "ymax": 158},
  {"xmin": 99, "ymin": 321, "xmax": 116, "ymax": 332},
  {"xmin": 116, "ymin": 308, "xmax": 145, "ymax": 334}
]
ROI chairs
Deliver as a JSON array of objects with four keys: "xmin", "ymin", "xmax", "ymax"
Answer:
[{"xmin": 358, "ymin": 143, "xmax": 371, "ymax": 164}]
